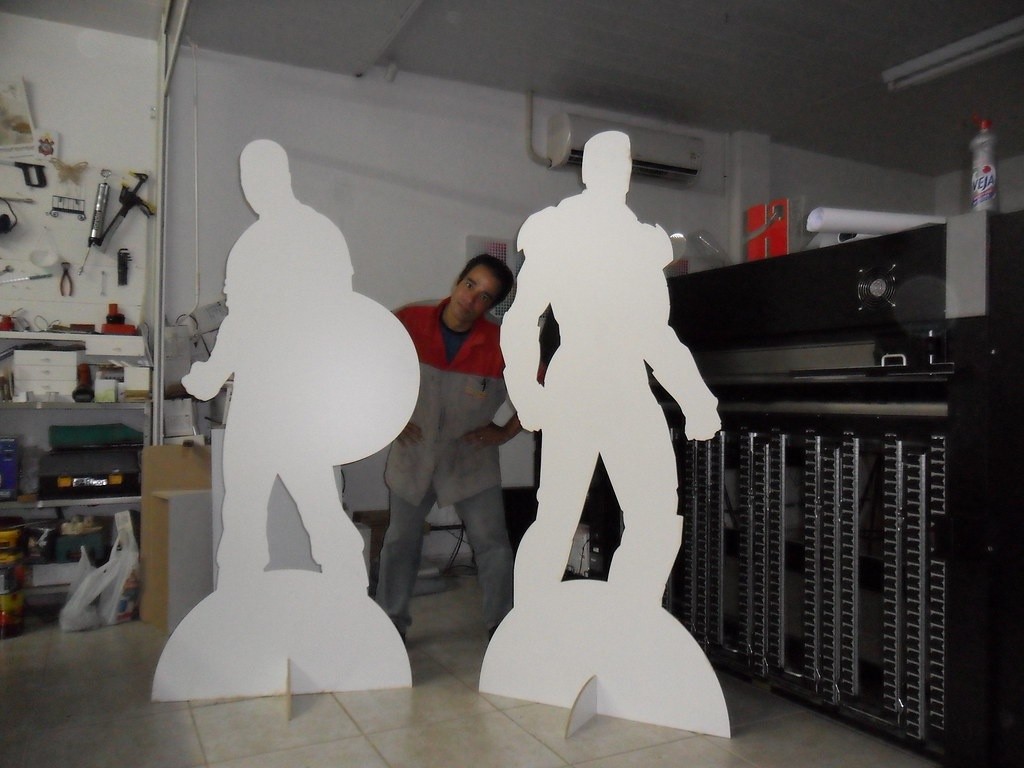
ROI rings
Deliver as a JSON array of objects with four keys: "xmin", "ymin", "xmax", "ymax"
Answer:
[{"xmin": 480, "ymin": 436, "xmax": 483, "ymax": 440}]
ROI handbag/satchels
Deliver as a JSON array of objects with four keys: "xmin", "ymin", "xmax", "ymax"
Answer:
[{"xmin": 58, "ymin": 511, "xmax": 141, "ymax": 632}]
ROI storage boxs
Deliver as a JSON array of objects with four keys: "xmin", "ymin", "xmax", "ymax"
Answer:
[
  {"xmin": 11, "ymin": 347, "xmax": 85, "ymax": 404},
  {"xmin": 164, "ymin": 398, "xmax": 199, "ymax": 437},
  {"xmin": 15, "ymin": 564, "xmax": 78, "ymax": 587}
]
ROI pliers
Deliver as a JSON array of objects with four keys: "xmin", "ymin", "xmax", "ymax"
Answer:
[{"xmin": 60, "ymin": 262, "xmax": 73, "ymax": 296}]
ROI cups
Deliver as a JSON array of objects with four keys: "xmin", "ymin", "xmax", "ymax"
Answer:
[{"xmin": 123, "ymin": 367, "xmax": 150, "ymax": 400}]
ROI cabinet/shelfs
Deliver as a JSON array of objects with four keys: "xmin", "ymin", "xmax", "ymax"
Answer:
[{"xmin": 0, "ymin": 327, "xmax": 151, "ymax": 610}]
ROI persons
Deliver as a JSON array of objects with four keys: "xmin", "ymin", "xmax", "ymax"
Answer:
[{"xmin": 374, "ymin": 253, "xmax": 546, "ymax": 646}]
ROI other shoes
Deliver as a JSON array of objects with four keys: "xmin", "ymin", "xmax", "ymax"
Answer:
[
  {"xmin": 487, "ymin": 626, "xmax": 498, "ymax": 640},
  {"xmin": 401, "ymin": 633, "xmax": 406, "ymax": 645}
]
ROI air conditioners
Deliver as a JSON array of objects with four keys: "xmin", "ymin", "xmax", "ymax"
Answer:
[
  {"xmin": 880, "ymin": 17, "xmax": 1024, "ymax": 95},
  {"xmin": 546, "ymin": 112, "xmax": 706, "ymax": 189}
]
